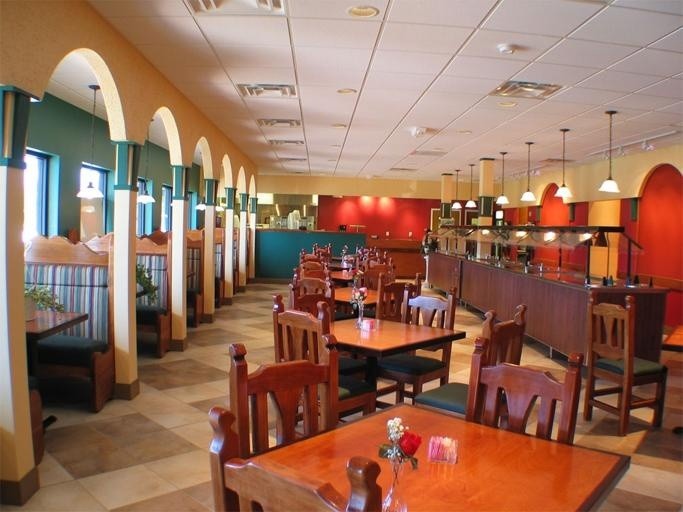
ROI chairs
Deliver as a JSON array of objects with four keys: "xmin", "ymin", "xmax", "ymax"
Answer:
[
  {"xmin": 301, "ymin": 248, "xmax": 323, "ymax": 262},
  {"xmin": 464, "ymin": 336, "xmax": 585, "ymax": 445},
  {"xmin": 375, "ymin": 272, "xmax": 423, "ymax": 323},
  {"xmin": 582, "ymin": 289, "xmax": 668, "ymax": 436},
  {"xmin": 414, "ymin": 303, "xmax": 529, "ymax": 419},
  {"xmin": 293, "ymin": 272, "xmax": 326, "ymax": 299},
  {"xmin": 365, "ymin": 259, "xmax": 393, "ymax": 288},
  {"xmin": 271, "ymin": 294, "xmax": 376, "ymax": 441},
  {"xmin": 376, "ymin": 282, "xmax": 457, "ymax": 410},
  {"xmin": 297, "ymin": 260, "xmax": 324, "ymax": 277},
  {"xmin": 228, "ymin": 334, "xmax": 339, "ymax": 459},
  {"xmin": 288, "ymin": 280, "xmax": 335, "ymax": 322},
  {"xmin": 313, "ymin": 243, "xmax": 332, "ymax": 263},
  {"xmin": 208, "ymin": 406, "xmax": 383, "ymax": 512}
]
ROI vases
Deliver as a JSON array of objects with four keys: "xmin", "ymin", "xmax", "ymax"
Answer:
[
  {"xmin": 354, "ymin": 303, "xmax": 365, "ymax": 329},
  {"xmin": 382, "ymin": 463, "xmax": 408, "ymax": 512},
  {"xmin": 353, "ymin": 279, "xmax": 358, "ymax": 289}
]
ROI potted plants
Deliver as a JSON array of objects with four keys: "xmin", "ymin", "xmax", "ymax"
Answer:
[
  {"xmin": 417, "ymin": 237, "xmax": 439, "ymax": 256},
  {"xmin": 24, "ymin": 284, "xmax": 65, "ymax": 325}
]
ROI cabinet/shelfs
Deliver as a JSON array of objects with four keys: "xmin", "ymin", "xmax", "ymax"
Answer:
[
  {"xmin": 428, "ymin": 251, "xmax": 462, "ymax": 301},
  {"xmin": 368, "ymin": 237, "xmax": 427, "ymax": 279},
  {"xmin": 460, "ymin": 255, "xmax": 672, "ymax": 368}
]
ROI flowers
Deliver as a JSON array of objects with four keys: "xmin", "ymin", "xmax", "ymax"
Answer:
[
  {"xmin": 378, "ymin": 417, "xmax": 422, "ymax": 489},
  {"xmin": 350, "ymin": 287, "xmax": 368, "ymax": 311},
  {"xmin": 351, "ymin": 269, "xmax": 365, "ymax": 279}
]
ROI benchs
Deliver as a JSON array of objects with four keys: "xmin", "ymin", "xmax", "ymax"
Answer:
[
  {"xmin": 24, "ymin": 234, "xmax": 115, "ymax": 411},
  {"xmin": 69, "ymin": 230, "xmax": 172, "ymax": 359},
  {"xmin": 164, "ymin": 228, "xmax": 225, "ymax": 309},
  {"xmin": 139, "ymin": 230, "xmax": 205, "ymax": 328}
]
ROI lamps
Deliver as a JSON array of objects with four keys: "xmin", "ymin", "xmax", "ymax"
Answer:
[
  {"xmin": 194, "ymin": 152, "xmax": 207, "ymax": 211},
  {"xmin": 554, "ymin": 127, "xmax": 573, "ymax": 199},
  {"xmin": 137, "ymin": 118, "xmax": 157, "ymax": 204},
  {"xmin": 76, "ymin": 83, "xmax": 106, "ymax": 200},
  {"xmin": 598, "ymin": 110, "xmax": 621, "ymax": 194},
  {"xmin": 451, "ymin": 169, "xmax": 463, "ymax": 208},
  {"xmin": 495, "ymin": 151, "xmax": 510, "ymax": 204},
  {"xmin": 520, "ymin": 141, "xmax": 537, "ymax": 202},
  {"xmin": 464, "ymin": 163, "xmax": 478, "ymax": 208}
]
